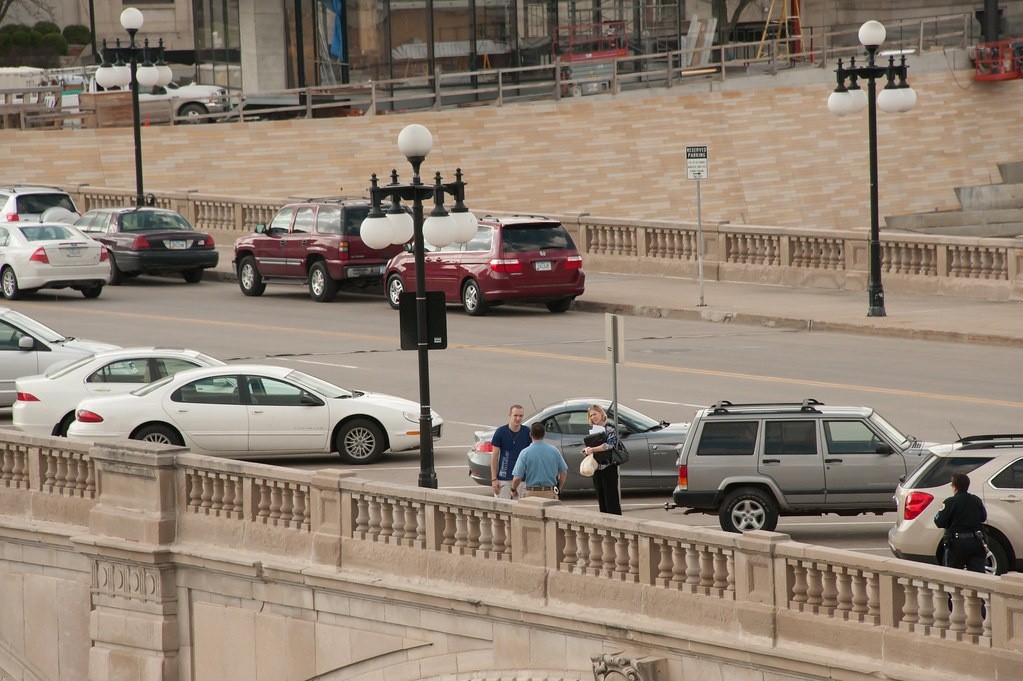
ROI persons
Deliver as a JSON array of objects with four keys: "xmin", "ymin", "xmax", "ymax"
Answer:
[
  {"xmin": 493, "ymin": 405, "xmax": 568, "ymax": 500},
  {"xmin": 580, "ymin": 405, "xmax": 621, "ymax": 516},
  {"xmin": 934, "ymin": 473, "xmax": 987, "ymax": 573}
]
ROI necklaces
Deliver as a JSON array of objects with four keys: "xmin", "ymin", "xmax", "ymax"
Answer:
[{"xmin": 509, "ymin": 427, "xmax": 519, "ymax": 444}]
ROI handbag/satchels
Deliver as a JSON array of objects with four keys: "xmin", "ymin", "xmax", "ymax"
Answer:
[
  {"xmin": 580, "ymin": 453, "xmax": 599, "ymax": 477},
  {"xmin": 606, "ymin": 428, "xmax": 630, "ymax": 466}
]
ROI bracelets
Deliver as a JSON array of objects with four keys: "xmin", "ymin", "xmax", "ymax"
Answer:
[
  {"xmin": 511, "ymin": 487, "xmax": 516, "ymax": 493},
  {"xmin": 592, "ymin": 447, "xmax": 597, "ymax": 454},
  {"xmin": 491, "ymin": 479, "xmax": 497, "ymax": 482}
]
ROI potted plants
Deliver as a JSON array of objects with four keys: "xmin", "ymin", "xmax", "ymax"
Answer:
[{"xmin": 0, "ymin": 19, "xmax": 91, "ymax": 69}]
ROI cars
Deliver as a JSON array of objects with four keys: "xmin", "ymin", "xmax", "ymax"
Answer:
[
  {"xmin": 0, "ymin": 306, "xmax": 124, "ymax": 414},
  {"xmin": 0, "ymin": 222, "xmax": 114, "ymax": 301},
  {"xmin": 466, "ymin": 398, "xmax": 691, "ymax": 495},
  {"xmin": 66, "ymin": 362, "xmax": 448, "ymax": 466},
  {"xmin": 11, "ymin": 344, "xmax": 230, "ymax": 439},
  {"xmin": 74, "ymin": 209, "xmax": 218, "ymax": 286}
]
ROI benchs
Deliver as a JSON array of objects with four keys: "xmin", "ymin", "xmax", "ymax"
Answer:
[{"xmin": 0, "ymin": 86, "xmax": 63, "ymax": 129}]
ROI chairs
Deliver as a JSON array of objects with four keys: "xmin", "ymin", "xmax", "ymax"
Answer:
[
  {"xmin": 37, "ymin": 230, "xmax": 52, "ymax": 240},
  {"xmin": 250, "ymin": 393, "xmax": 258, "ymax": 404},
  {"xmin": 557, "ymin": 412, "xmax": 574, "ymax": 439},
  {"xmin": 157, "ymin": 361, "xmax": 169, "ymax": 378},
  {"xmin": 181, "ymin": 384, "xmax": 197, "ymax": 402},
  {"xmin": 232, "ymin": 387, "xmax": 240, "ymax": 404},
  {"xmin": 92, "ymin": 373, "xmax": 104, "ymax": 383},
  {"xmin": 144, "ymin": 363, "xmax": 151, "ymax": 383}
]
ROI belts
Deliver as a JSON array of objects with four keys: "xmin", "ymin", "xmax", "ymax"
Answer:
[
  {"xmin": 527, "ymin": 487, "xmax": 555, "ymax": 491},
  {"xmin": 957, "ymin": 532, "xmax": 976, "ymax": 538}
]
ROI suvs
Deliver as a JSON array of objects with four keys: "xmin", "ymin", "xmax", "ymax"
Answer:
[
  {"xmin": 87, "ymin": 73, "xmax": 229, "ymax": 125},
  {"xmin": 663, "ymin": 398, "xmax": 944, "ymax": 535},
  {"xmin": 233, "ymin": 196, "xmax": 414, "ymax": 304},
  {"xmin": 383, "ymin": 213, "xmax": 585, "ymax": 317},
  {"xmin": 887, "ymin": 433, "xmax": 1023, "ymax": 577},
  {"xmin": 1, "ymin": 184, "xmax": 82, "ymax": 226}
]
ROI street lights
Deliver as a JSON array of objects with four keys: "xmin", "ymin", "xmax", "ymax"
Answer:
[
  {"xmin": 358, "ymin": 123, "xmax": 479, "ymax": 492},
  {"xmin": 94, "ymin": 8, "xmax": 173, "ymax": 208},
  {"xmin": 828, "ymin": 19, "xmax": 917, "ymax": 319}
]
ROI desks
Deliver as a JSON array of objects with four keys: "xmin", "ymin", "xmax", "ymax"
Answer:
[{"xmin": 393, "ymin": 39, "xmax": 511, "ymax": 77}]
